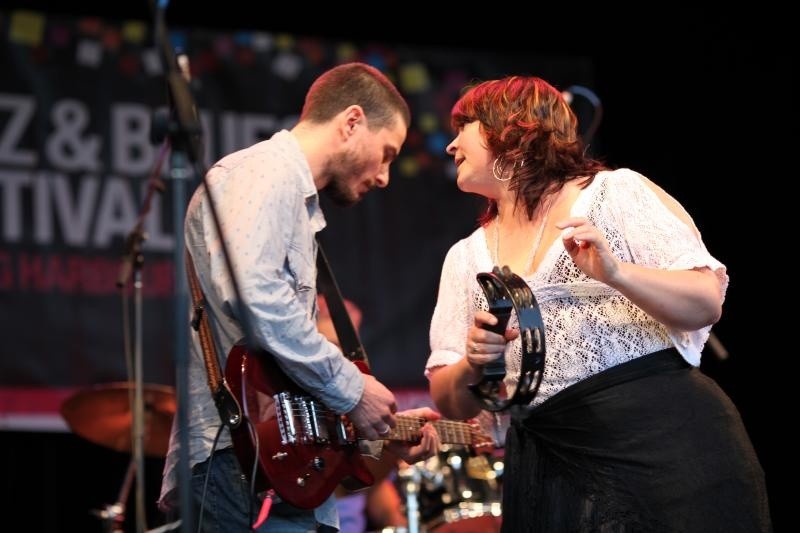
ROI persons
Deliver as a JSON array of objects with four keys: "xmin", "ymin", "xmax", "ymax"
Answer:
[
  {"xmin": 424, "ymin": 77, "xmax": 770, "ymax": 533},
  {"xmin": 159, "ymin": 63, "xmax": 443, "ymax": 533}
]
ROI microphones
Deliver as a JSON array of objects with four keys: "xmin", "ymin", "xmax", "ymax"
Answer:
[{"xmin": 172, "ymin": 51, "xmax": 201, "ymax": 151}]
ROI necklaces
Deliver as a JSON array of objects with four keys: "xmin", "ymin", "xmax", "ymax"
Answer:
[{"xmin": 493, "ymin": 189, "xmax": 558, "ymax": 278}]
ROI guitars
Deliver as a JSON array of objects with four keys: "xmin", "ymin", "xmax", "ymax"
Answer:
[{"xmin": 225, "ymin": 336, "xmax": 498, "ymax": 519}]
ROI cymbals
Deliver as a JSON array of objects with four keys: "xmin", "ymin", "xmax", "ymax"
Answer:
[
  {"xmin": 61, "ymin": 381, "xmax": 181, "ymax": 456},
  {"xmin": 464, "ymin": 263, "xmax": 547, "ymax": 415}
]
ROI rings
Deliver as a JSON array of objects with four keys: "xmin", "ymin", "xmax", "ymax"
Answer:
[{"xmin": 379, "ymin": 425, "xmax": 390, "ymax": 434}]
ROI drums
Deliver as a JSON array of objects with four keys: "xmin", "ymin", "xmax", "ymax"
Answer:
[{"xmin": 414, "ymin": 453, "xmax": 502, "ymax": 532}]
